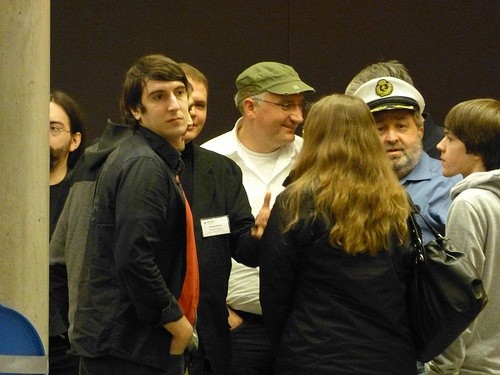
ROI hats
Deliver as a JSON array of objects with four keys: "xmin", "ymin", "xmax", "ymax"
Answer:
[
  {"xmin": 350, "ymin": 75, "xmax": 426, "ymax": 115},
  {"xmin": 235, "ymin": 61, "xmax": 316, "ymax": 105}
]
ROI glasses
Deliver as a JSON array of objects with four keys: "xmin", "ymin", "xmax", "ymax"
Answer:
[
  {"xmin": 50, "ymin": 124, "xmax": 74, "ymax": 135},
  {"xmin": 252, "ymin": 96, "xmax": 309, "ymax": 111}
]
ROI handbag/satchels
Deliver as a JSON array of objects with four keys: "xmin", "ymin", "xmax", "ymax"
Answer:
[{"xmin": 405, "ymin": 197, "xmax": 489, "ymax": 364}]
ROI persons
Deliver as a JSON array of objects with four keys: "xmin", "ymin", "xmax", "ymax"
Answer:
[
  {"xmin": 258, "ymin": 93, "xmax": 422, "ymax": 375},
  {"xmin": 342, "ymin": 60, "xmax": 500, "ymax": 375},
  {"xmin": 48, "ymin": 54, "xmax": 271, "ymax": 375},
  {"xmin": 200, "ymin": 60, "xmax": 317, "ymax": 375}
]
